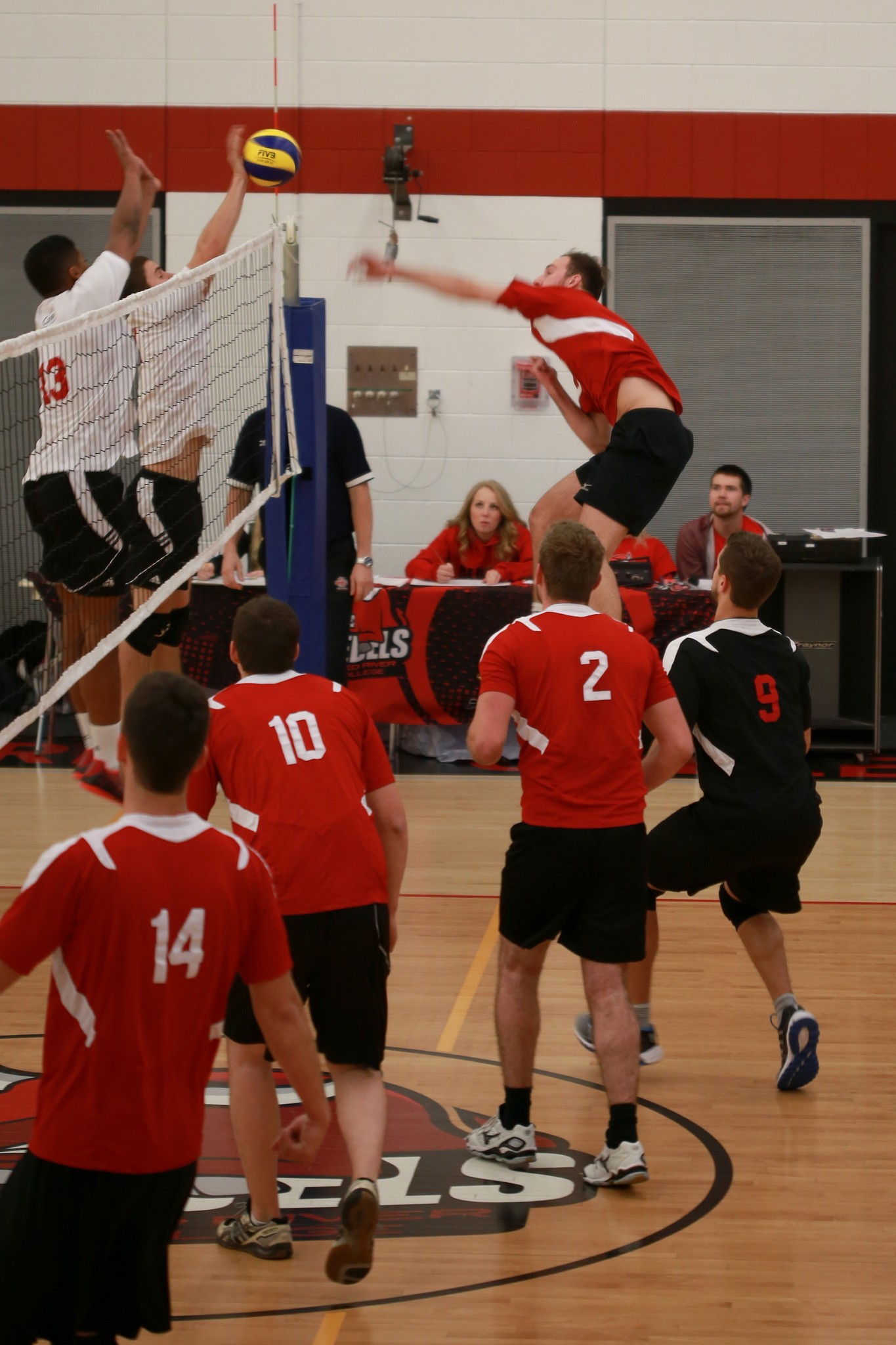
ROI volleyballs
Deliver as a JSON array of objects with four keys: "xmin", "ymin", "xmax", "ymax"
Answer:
[{"xmin": 241, "ymin": 129, "xmax": 301, "ymax": 187}]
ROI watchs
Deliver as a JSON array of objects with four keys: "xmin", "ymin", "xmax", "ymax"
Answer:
[{"xmin": 354, "ymin": 555, "xmax": 372, "ymax": 568}]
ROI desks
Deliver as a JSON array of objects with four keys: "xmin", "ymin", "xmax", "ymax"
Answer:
[{"xmin": 17, "ymin": 568, "xmax": 719, "ymax": 767}]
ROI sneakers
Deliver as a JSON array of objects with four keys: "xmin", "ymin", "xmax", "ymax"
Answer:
[
  {"xmin": 572, "ymin": 1009, "xmax": 663, "ymax": 1064},
  {"xmin": 463, "ymin": 1103, "xmax": 537, "ymax": 1164},
  {"xmin": 769, "ymin": 1004, "xmax": 820, "ymax": 1091},
  {"xmin": 324, "ymin": 1177, "xmax": 379, "ymax": 1285},
  {"xmin": 216, "ymin": 1200, "xmax": 293, "ymax": 1260},
  {"xmin": 75, "ymin": 749, "xmax": 124, "ymax": 807},
  {"xmin": 583, "ymin": 1140, "xmax": 649, "ymax": 1186}
]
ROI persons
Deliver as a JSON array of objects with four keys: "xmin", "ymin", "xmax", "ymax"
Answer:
[
  {"xmin": 675, "ymin": 463, "xmax": 770, "ymax": 583},
  {"xmin": 185, "ymin": 595, "xmax": 408, "ymax": 1283},
  {"xmin": 221, "ymin": 404, "xmax": 373, "ymax": 687},
  {"xmin": 575, "ymin": 531, "xmax": 823, "ymax": 1092},
  {"xmin": 465, "ymin": 518, "xmax": 694, "ymax": 1188},
  {"xmin": 197, "ymin": 517, "xmax": 255, "ymax": 582},
  {"xmin": 21, "ymin": 130, "xmax": 161, "ymax": 807},
  {"xmin": 404, "ymin": 480, "xmax": 534, "ymax": 585},
  {"xmin": 608, "ymin": 529, "xmax": 676, "ymax": 591},
  {"xmin": 345, "ymin": 252, "xmax": 694, "ymax": 624},
  {"xmin": 120, "ymin": 125, "xmax": 246, "ymax": 723},
  {"xmin": 0, "ymin": 668, "xmax": 333, "ymax": 1345}
]
